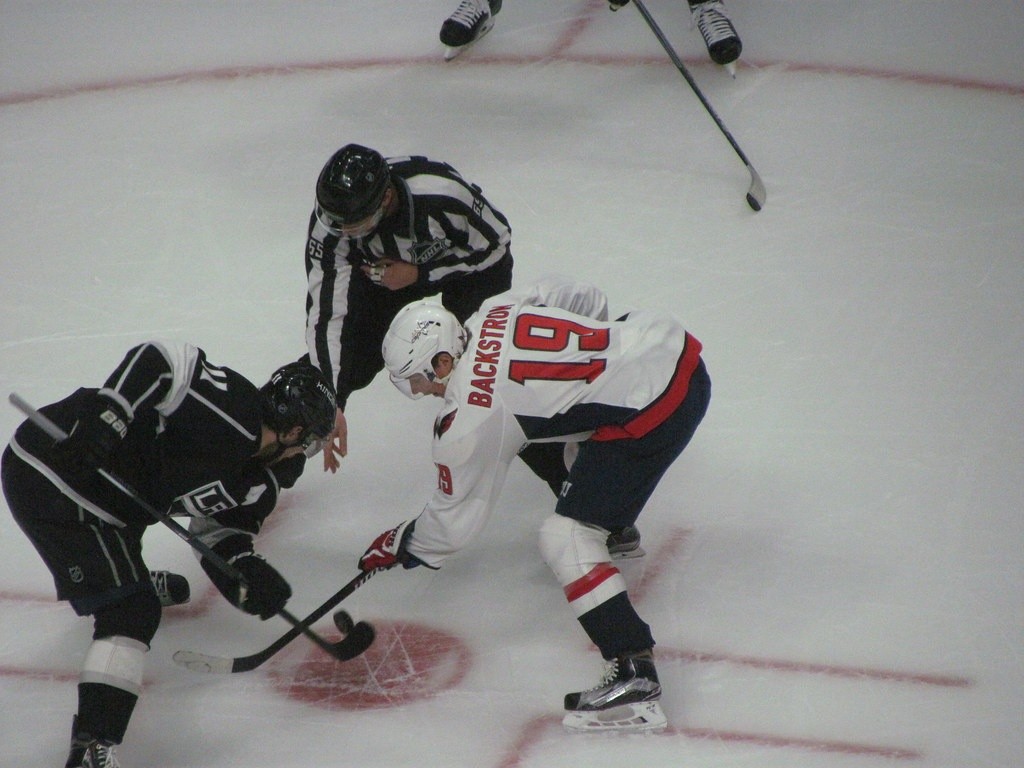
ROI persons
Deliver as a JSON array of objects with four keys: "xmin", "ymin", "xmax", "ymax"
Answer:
[
  {"xmin": 358, "ymin": 272, "xmax": 711, "ymax": 734},
  {"xmin": 304, "ymin": 143, "xmax": 513, "ymax": 475},
  {"xmin": 439, "ymin": 0, "xmax": 742, "ymax": 79},
  {"xmin": 0, "ymin": 339, "xmax": 336, "ymax": 768}
]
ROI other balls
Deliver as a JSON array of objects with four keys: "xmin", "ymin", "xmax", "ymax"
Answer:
[{"xmin": 333, "ymin": 610, "xmax": 355, "ymax": 633}]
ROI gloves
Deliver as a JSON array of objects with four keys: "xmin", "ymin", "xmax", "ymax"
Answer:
[
  {"xmin": 201, "ymin": 534, "xmax": 292, "ymax": 620},
  {"xmin": 54, "ymin": 394, "xmax": 128, "ymax": 475},
  {"xmin": 358, "ymin": 519, "xmax": 421, "ymax": 572}
]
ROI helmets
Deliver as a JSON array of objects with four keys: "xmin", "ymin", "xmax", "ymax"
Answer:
[
  {"xmin": 269, "ymin": 361, "xmax": 337, "ymax": 439},
  {"xmin": 381, "ymin": 300, "xmax": 467, "ymax": 378},
  {"xmin": 316, "ymin": 143, "xmax": 391, "ymax": 225}
]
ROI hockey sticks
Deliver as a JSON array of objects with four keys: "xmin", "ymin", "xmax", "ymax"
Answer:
[
  {"xmin": 169, "ymin": 567, "xmax": 383, "ymax": 673},
  {"xmin": 636, "ymin": 0, "xmax": 767, "ymax": 211},
  {"xmin": 9, "ymin": 391, "xmax": 375, "ymax": 659}
]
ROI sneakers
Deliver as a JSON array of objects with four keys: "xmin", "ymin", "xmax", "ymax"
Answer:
[
  {"xmin": 605, "ymin": 525, "xmax": 647, "ymax": 559},
  {"xmin": 149, "ymin": 570, "xmax": 191, "ymax": 607},
  {"xmin": 65, "ymin": 713, "xmax": 123, "ymax": 768},
  {"xmin": 439, "ymin": 0, "xmax": 502, "ymax": 63},
  {"xmin": 562, "ymin": 648, "xmax": 667, "ymax": 734},
  {"xmin": 689, "ymin": 0, "xmax": 742, "ymax": 80}
]
